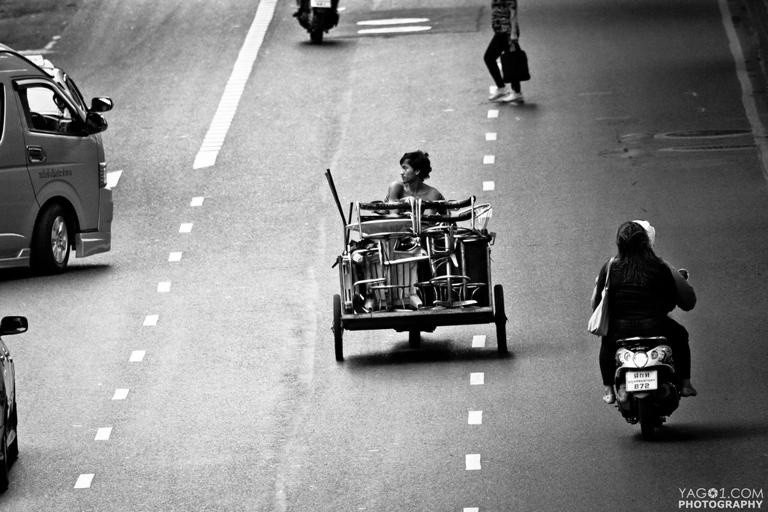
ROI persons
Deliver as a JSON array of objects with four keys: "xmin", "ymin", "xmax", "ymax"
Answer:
[
  {"xmin": 380, "ymin": 149, "xmax": 457, "ymax": 306},
  {"xmin": 592, "ymin": 220, "xmax": 699, "ymax": 404},
  {"xmin": 290, "ymin": 0, "xmax": 342, "ymax": 28},
  {"xmin": 587, "ymin": 218, "xmax": 698, "ymax": 312},
  {"xmin": 483, "ymin": 0, "xmax": 523, "ymax": 103}
]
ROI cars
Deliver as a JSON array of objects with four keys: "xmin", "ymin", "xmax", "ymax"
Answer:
[{"xmin": 1, "ymin": 316, "xmax": 29, "ymax": 495}]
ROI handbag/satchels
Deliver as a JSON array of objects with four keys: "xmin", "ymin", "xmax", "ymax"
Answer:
[
  {"xmin": 501, "ymin": 39, "xmax": 529, "ymax": 83},
  {"xmin": 587, "ymin": 256, "xmax": 614, "ymax": 337}
]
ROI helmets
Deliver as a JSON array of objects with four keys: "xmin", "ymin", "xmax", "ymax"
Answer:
[
  {"xmin": 615, "ymin": 222, "xmax": 649, "ymax": 249},
  {"xmin": 631, "ymin": 219, "xmax": 655, "ymax": 247}
]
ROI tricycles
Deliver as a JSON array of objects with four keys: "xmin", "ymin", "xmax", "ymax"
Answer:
[{"xmin": 331, "ymin": 196, "xmax": 508, "ymax": 364}]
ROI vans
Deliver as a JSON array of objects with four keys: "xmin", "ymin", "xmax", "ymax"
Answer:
[{"xmin": 1, "ymin": 41, "xmax": 113, "ymax": 270}]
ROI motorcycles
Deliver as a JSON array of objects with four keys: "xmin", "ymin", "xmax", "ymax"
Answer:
[
  {"xmin": 297, "ymin": 1, "xmax": 337, "ymax": 42},
  {"xmin": 611, "ymin": 270, "xmax": 690, "ymax": 440}
]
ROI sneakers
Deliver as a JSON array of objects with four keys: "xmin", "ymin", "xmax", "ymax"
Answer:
[
  {"xmin": 489, "ymin": 85, "xmax": 511, "ymax": 100},
  {"xmin": 502, "ymin": 91, "xmax": 524, "ymax": 103}
]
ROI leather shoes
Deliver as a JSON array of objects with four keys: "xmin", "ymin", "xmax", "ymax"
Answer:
[
  {"xmin": 679, "ymin": 385, "xmax": 696, "ymax": 397},
  {"xmin": 602, "ymin": 394, "xmax": 615, "ymax": 404}
]
